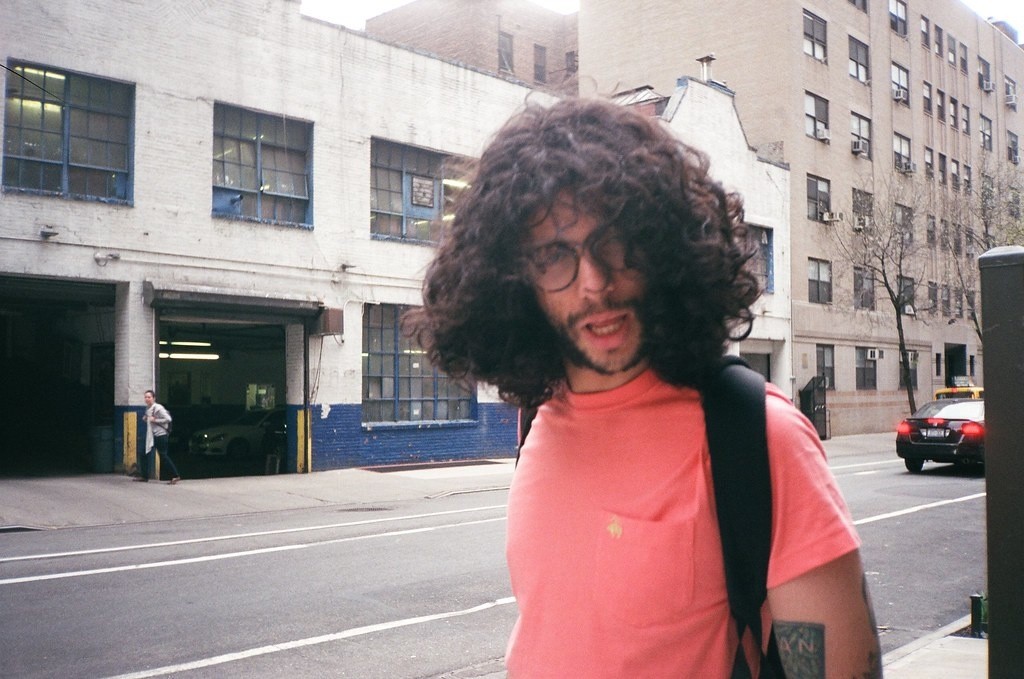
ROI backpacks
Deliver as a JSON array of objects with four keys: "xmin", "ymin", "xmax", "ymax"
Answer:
[{"xmin": 166, "ymin": 422, "xmax": 172, "ymax": 434}]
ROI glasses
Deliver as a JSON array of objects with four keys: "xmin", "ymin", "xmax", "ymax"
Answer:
[{"xmin": 524, "ymin": 234, "xmax": 637, "ymax": 294}]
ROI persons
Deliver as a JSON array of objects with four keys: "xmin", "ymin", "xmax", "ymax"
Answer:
[
  {"xmin": 134, "ymin": 390, "xmax": 181, "ymax": 485},
  {"xmin": 400, "ymin": 96, "xmax": 881, "ymax": 679}
]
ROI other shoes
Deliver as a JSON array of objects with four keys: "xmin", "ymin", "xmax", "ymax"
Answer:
[
  {"xmin": 133, "ymin": 476, "xmax": 145, "ymax": 482},
  {"xmin": 167, "ymin": 476, "xmax": 180, "ymax": 485}
]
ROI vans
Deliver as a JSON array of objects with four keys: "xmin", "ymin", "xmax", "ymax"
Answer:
[{"xmin": 933, "ymin": 387, "xmax": 985, "ymax": 401}]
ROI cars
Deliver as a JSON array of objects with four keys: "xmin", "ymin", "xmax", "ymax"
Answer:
[
  {"xmin": 892, "ymin": 397, "xmax": 986, "ymax": 475},
  {"xmin": 189, "ymin": 407, "xmax": 282, "ymax": 464}
]
